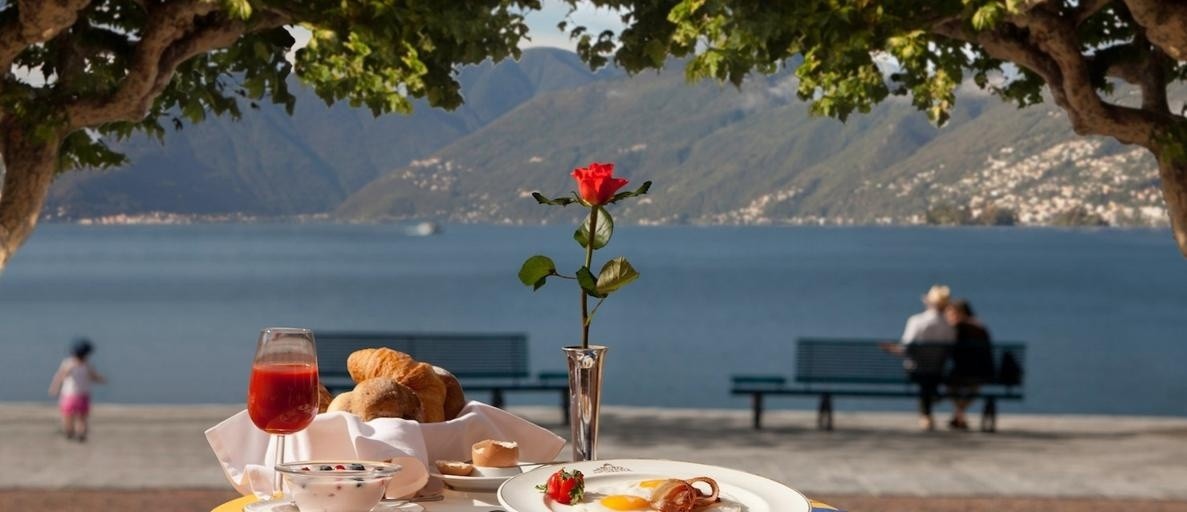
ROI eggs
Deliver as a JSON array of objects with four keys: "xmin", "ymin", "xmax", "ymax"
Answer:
[
  {"xmin": 586, "ymin": 480, "xmax": 669, "ymax": 512},
  {"xmin": 435, "ymin": 439, "xmax": 519, "ymax": 476}
]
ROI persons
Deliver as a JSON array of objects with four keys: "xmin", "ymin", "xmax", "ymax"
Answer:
[
  {"xmin": 46, "ymin": 336, "xmax": 104, "ymax": 441},
  {"xmin": 944, "ymin": 302, "xmax": 992, "ymax": 431},
  {"xmin": 897, "ymin": 284, "xmax": 956, "ymax": 432}
]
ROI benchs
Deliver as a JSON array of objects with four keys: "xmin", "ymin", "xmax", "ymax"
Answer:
[
  {"xmin": 731, "ymin": 335, "xmax": 1028, "ymax": 433},
  {"xmin": 289, "ymin": 331, "xmax": 572, "ymax": 423}
]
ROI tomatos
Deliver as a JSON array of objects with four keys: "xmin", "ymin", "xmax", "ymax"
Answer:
[{"xmin": 547, "ymin": 470, "xmax": 584, "ymax": 504}]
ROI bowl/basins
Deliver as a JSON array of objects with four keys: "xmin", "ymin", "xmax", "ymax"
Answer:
[{"xmin": 278, "ymin": 460, "xmax": 402, "ymax": 512}]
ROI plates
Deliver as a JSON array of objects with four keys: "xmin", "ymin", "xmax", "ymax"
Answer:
[
  {"xmin": 432, "ymin": 461, "xmax": 548, "ymax": 490},
  {"xmin": 498, "ymin": 459, "xmax": 809, "ymax": 511},
  {"xmin": 242, "ymin": 491, "xmax": 428, "ymax": 511}
]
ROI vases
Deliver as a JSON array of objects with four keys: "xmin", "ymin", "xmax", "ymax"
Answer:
[{"xmin": 561, "ymin": 345, "xmax": 608, "ymax": 464}]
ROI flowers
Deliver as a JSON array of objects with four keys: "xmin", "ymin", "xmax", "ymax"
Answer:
[{"xmin": 517, "ymin": 161, "xmax": 652, "ymax": 349}]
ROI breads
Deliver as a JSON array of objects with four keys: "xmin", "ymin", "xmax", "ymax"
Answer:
[{"xmin": 317, "ymin": 347, "xmax": 465, "ymax": 423}]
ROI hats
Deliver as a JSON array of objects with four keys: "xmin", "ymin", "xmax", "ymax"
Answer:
[{"xmin": 918, "ymin": 279, "xmax": 954, "ymax": 312}]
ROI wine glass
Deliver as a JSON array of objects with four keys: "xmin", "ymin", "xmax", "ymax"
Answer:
[{"xmin": 248, "ymin": 327, "xmax": 319, "ymax": 492}]
